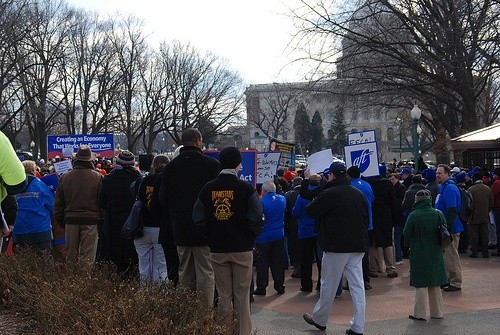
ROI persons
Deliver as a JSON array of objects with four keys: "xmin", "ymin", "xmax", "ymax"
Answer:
[
  {"xmin": 55, "ymin": 146, "xmax": 104, "ymax": 269},
  {"xmin": 435, "ymin": 163, "xmax": 463, "ymax": 291},
  {"xmin": 304, "ymin": 162, "xmax": 370, "ymax": 335},
  {"xmin": 18, "ymin": 155, "xmax": 77, "ymax": 179},
  {"xmin": 92, "ymin": 156, "xmax": 118, "ymax": 176},
  {"xmin": 128, "ymin": 154, "xmax": 171, "ymax": 283},
  {"xmin": 96, "ymin": 150, "xmax": 142, "ymax": 280},
  {"xmin": 190, "ymin": 146, "xmax": 263, "ymax": 335},
  {"xmin": 249, "ymin": 162, "xmax": 500, "ymax": 302},
  {"xmin": 0, "ymin": 187, "xmax": 18, "ymax": 256},
  {"xmin": 402, "ymin": 189, "xmax": 447, "ymax": 322},
  {"xmin": 157, "ymin": 128, "xmax": 223, "ymax": 320},
  {"xmin": 50, "ymin": 216, "xmax": 67, "ymax": 252},
  {"xmin": 10, "ymin": 159, "xmax": 55, "ymax": 255}
]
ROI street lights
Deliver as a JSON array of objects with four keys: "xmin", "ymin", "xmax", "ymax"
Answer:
[
  {"xmin": 397, "ymin": 117, "xmax": 404, "ymax": 160},
  {"xmin": 410, "ymin": 99, "xmax": 422, "ymax": 174}
]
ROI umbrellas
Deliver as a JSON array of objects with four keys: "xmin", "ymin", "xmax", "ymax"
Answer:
[{"xmin": 96, "ymin": 150, "xmax": 121, "ymax": 159}]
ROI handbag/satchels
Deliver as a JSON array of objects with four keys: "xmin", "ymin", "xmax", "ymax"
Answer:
[
  {"xmin": 437, "ymin": 211, "xmax": 454, "ymax": 248},
  {"xmin": 122, "ymin": 175, "xmax": 146, "ymax": 239}
]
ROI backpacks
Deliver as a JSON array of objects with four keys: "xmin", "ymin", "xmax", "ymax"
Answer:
[{"xmin": 443, "ymin": 183, "xmax": 475, "ymax": 225}]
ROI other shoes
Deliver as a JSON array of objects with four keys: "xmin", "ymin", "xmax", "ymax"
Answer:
[
  {"xmin": 482, "ymin": 253, "xmax": 489, "ymax": 258},
  {"xmin": 253, "ymin": 260, "xmax": 404, "ymax": 298},
  {"xmin": 470, "ymin": 253, "xmax": 478, "ymax": 258},
  {"xmin": 303, "ymin": 313, "xmax": 327, "ymax": 330},
  {"xmin": 409, "ymin": 315, "xmax": 426, "ymax": 321},
  {"xmin": 345, "ymin": 328, "xmax": 363, "ymax": 335},
  {"xmin": 440, "ymin": 284, "xmax": 461, "ymax": 291}
]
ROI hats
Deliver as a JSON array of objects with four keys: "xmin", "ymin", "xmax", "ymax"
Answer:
[
  {"xmin": 421, "ymin": 169, "xmax": 436, "ymax": 181},
  {"xmin": 324, "ymin": 162, "xmax": 345, "ymax": 174},
  {"xmin": 398, "ymin": 168, "xmax": 413, "ymax": 175},
  {"xmin": 455, "ymin": 171, "xmax": 466, "ymax": 182},
  {"xmin": 72, "ymin": 145, "xmax": 97, "ymax": 161},
  {"xmin": 415, "ymin": 190, "xmax": 431, "ymax": 203},
  {"xmin": 220, "ymin": 146, "xmax": 242, "ymax": 169},
  {"xmin": 116, "ymin": 150, "xmax": 136, "ymax": 166},
  {"xmin": 450, "ymin": 167, "xmax": 461, "ymax": 172},
  {"xmin": 348, "ymin": 165, "xmax": 360, "ymax": 178}
]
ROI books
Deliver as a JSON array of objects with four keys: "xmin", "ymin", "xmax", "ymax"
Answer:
[{"xmin": 40, "ymin": 173, "xmax": 59, "ymax": 195}]
